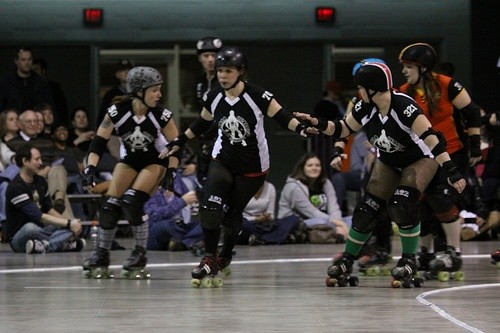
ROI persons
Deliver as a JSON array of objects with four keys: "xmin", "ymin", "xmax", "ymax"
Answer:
[
  {"xmin": 4, "ymin": 143, "xmax": 86, "ymax": 254},
  {"xmin": 236, "ymin": 178, "xmax": 298, "ymax": 246},
  {"xmin": 81, "ymin": 66, "xmax": 180, "ymax": 282},
  {"xmin": 293, "ymin": 42, "xmax": 500, "ymax": 289},
  {"xmin": 145, "ymin": 147, "xmax": 206, "ymax": 257},
  {"xmin": 277, "ymin": 153, "xmax": 350, "ymax": 245},
  {"xmin": 0, "ymin": 47, "xmax": 118, "ymax": 222},
  {"xmin": 179, "ymin": 36, "xmax": 228, "ymax": 257},
  {"xmin": 95, "ymin": 58, "xmax": 134, "ymax": 161},
  {"xmin": 156, "ymin": 46, "xmax": 319, "ymax": 288}
]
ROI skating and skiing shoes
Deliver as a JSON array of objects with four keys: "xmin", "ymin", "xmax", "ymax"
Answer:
[
  {"xmin": 81, "ymin": 247, "xmax": 111, "ymax": 279},
  {"xmin": 326, "ymin": 252, "xmax": 359, "ymax": 287},
  {"xmin": 190, "ymin": 253, "xmax": 233, "ymax": 289},
  {"xmin": 121, "ymin": 245, "xmax": 151, "ymax": 279},
  {"xmin": 491, "ymin": 250, "xmax": 500, "ymax": 266},
  {"xmin": 358, "ymin": 250, "xmax": 394, "ymax": 275},
  {"xmin": 390, "ymin": 256, "xmax": 425, "ymax": 288},
  {"xmin": 426, "ymin": 246, "xmax": 464, "ymax": 281}
]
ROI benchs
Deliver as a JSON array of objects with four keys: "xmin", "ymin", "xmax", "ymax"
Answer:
[{"xmin": 67, "ymin": 188, "xmax": 129, "ymax": 236}]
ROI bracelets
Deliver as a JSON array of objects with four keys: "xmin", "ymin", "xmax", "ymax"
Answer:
[{"xmin": 67, "ymin": 219, "xmax": 71, "ymax": 229}]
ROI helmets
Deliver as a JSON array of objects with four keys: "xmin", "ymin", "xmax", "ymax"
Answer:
[
  {"xmin": 125, "ymin": 66, "xmax": 164, "ymax": 94},
  {"xmin": 399, "ymin": 42, "xmax": 437, "ymax": 70},
  {"xmin": 196, "ymin": 37, "xmax": 223, "ymax": 54},
  {"xmin": 352, "ymin": 58, "xmax": 393, "ymax": 93},
  {"xmin": 215, "ymin": 49, "xmax": 245, "ymax": 71}
]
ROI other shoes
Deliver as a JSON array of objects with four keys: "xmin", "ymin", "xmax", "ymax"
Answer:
[
  {"xmin": 53, "ymin": 199, "xmax": 65, "ymax": 213},
  {"xmin": 249, "ymin": 234, "xmax": 266, "ymax": 245},
  {"xmin": 192, "ymin": 240, "xmax": 204, "ymax": 252},
  {"xmin": 169, "ymin": 240, "xmax": 189, "ymax": 251},
  {"xmin": 218, "ymin": 237, "xmax": 223, "ymax": 247}
]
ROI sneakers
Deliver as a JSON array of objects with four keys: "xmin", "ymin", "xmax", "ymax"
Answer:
[
  {"xmin": 26, "ymin": 238, "xmax": 50, "ymax": 255},
  {"xmin": 60, "ymin": 238, "xmax": 86, "ymax": 251}
]
